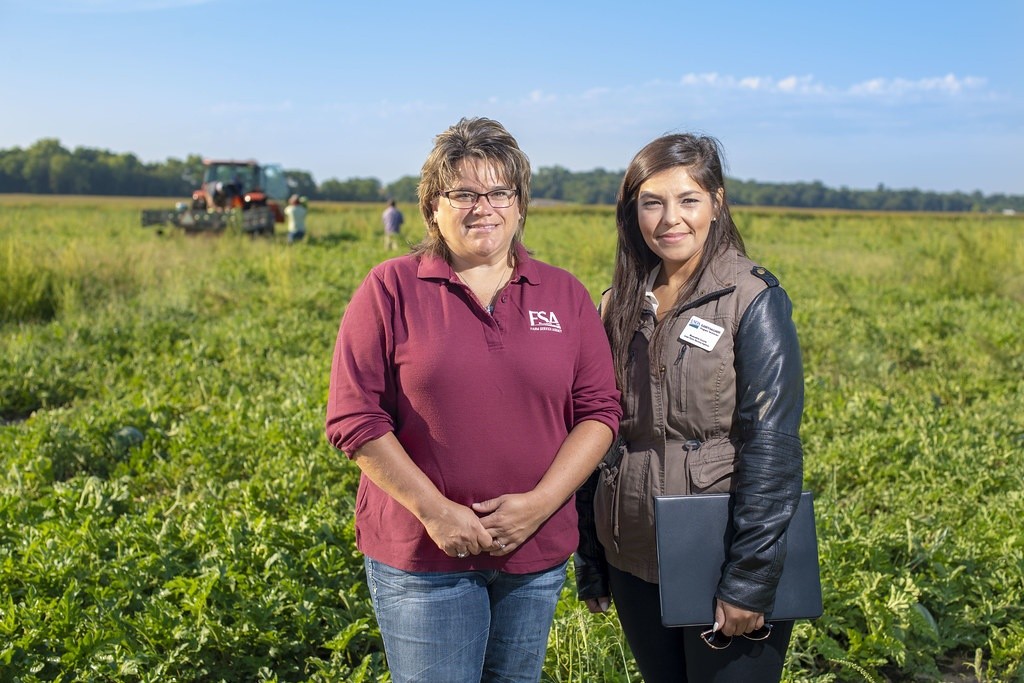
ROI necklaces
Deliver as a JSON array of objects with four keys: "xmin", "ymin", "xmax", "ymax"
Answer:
[{"xmin": 457, "ymin": 263, "xmax": 508, "ymax": 316}]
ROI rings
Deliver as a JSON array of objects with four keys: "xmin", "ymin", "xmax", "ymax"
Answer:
[
  {"xmin": 497, "ymin": 541, "xmax": 506, "ymax": 549},
  {"xmin": 457, "ymin": 550, "xmax": 469, "ymax": 558}
]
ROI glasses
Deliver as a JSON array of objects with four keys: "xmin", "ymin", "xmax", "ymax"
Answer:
[
  {"xmin": 433, "ymin": 188, "xmax": 520, "ymax": 209},
  {"xmin": 700, "ymin": 624, "xmax": 773, "ymax": 649}
]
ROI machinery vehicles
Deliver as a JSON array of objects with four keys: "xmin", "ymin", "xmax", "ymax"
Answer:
[{"xmin": 139, "ymin": 158, "xmax": 289, "ymax": 237}]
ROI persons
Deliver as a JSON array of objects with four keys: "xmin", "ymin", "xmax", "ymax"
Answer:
[
  {"xmin": 327, "ymin": 116, "xmax": 620, "ymax": 683},
  {"xmin": 203, "ymin": 173, "xmax": 404, "ymax": 251},
  {"xmin": 594, "ymin": 133, "xmax": 802, "ymax": 683}
]
